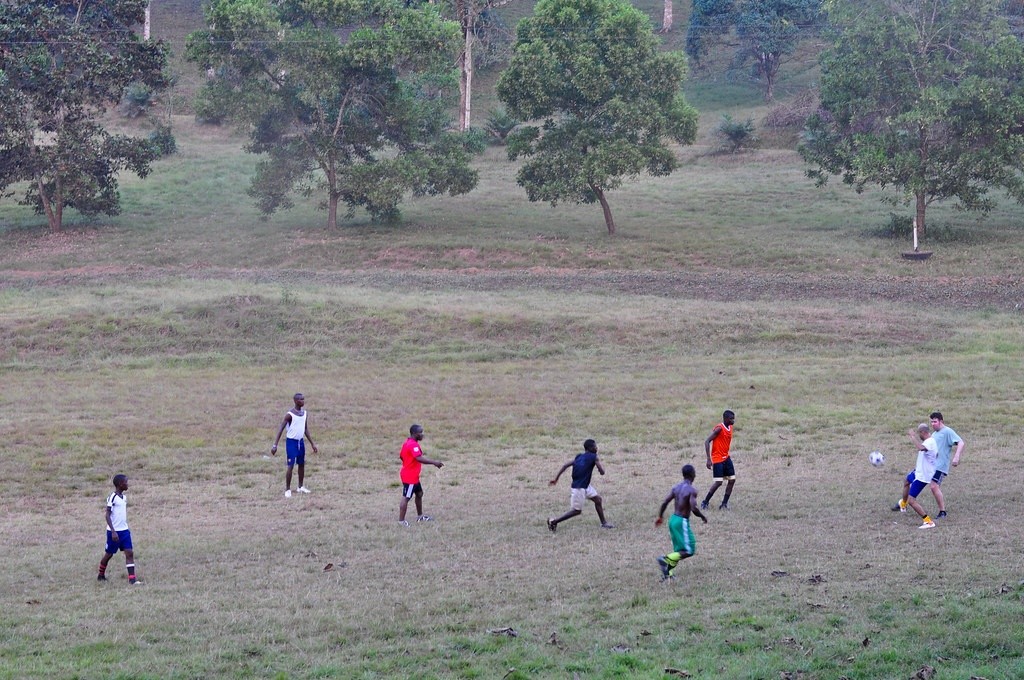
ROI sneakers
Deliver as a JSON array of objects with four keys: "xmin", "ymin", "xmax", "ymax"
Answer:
[
  {"xmin": 919, "ymin": 521, "xmax": 935, "ymax": 529},
  {"xmin": 898, "ymin": 499, "xmax": 906, "ymax": 512}
]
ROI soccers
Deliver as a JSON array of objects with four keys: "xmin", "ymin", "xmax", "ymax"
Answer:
[{"xmin": 870, "ymin": 452, "xmax": 885, "ymax": 466}]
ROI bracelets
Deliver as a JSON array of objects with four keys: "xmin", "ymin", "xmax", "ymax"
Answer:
[{"xmin": 273, "ymin": 445, "xmax": 277, "ymax": 447}]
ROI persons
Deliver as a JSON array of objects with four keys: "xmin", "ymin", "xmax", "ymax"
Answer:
[
  {"xmin": 271, "ymin": 394, "xmax": 318, "ymax": 497},
  {"xmin": 654, "ymin": 465, "xmax": 708, "ymax": 580},
  {"xmin": 899, "ymin": 423, "xmax": 936, "ymax": 529},
  {"xmin": 546, "ymin": 439, "xmax": 615, "ymax": 532},
  {"xmin": 97, "ymin": 475, "xmax": 142, "ymax": 586},
  {"xmin": 891, "ymin": 412, "xmax": 964, "ymax": 519},
  {"xmin": 701, "ymin": 410, "xmax": 736, "ymax": 510},
  {"xmin": 399, "ymin": 425, "xmax": 444, "ymax": 527}
]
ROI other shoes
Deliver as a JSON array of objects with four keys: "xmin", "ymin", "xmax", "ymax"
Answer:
[
  {"xmin": 936, "ymin": 512, "xmax": 947, "ymax": 519},
  {"xmin": 892, "ymin": 505, "xmax": 900, "ymax": 511},
  {"xmin": 658, "ymin": 556, "xmax": 675, "ymax": 575},
  {"xmin": 98, "ymin": 574, "xmax": 107, "ymax": 581},
  {"xmin": 398, "ymin": 520, "xmax": 410, "ymax": 528},
  {"xmin": 601, "ymin": 522, "xmax": 615, "ymax": 528},
  {"xmin": 701, "ymin": 500, "xmax": 709, "ymax": 510},
  {"xmin": 659, "ymin": 569, "xmax": 673, "ymax": 580},
  {"xmin": 128, "ymin": 578, "xmax": 141, "ymax": 585},
  {"xmin": 297, "ymin": 485, "xmax": 310, "ymax": 494},
  {"xmin": 285, "ymin": 490, "xmax": 291, "ymax": 498},
  {"xmin": 416, "ymin": 515, "xmax": 432, "ymax": 522},
  {"xmin": 719, "ymin": 505, "xmax": 729, "ymax": 511},
  {"xmin": 547, "ymin": 518, "xmax": 557, "ymax": 532}
]
